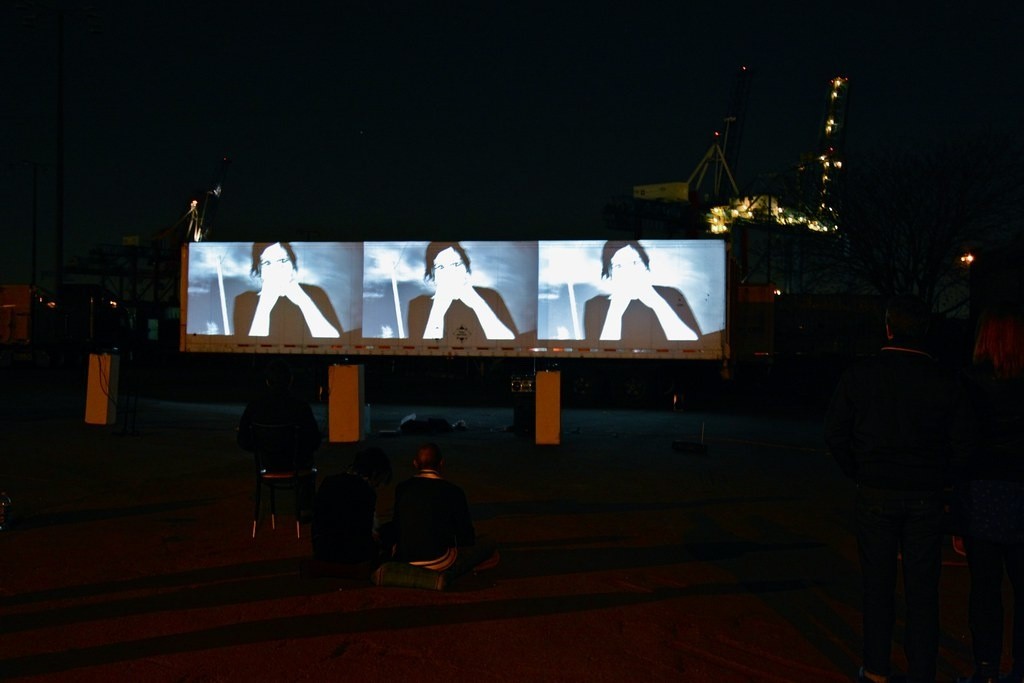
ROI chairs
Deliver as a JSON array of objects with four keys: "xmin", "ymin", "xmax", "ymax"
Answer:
[{"xmin": 248, "ymin": 423, "xmax": 317, "ymax": 540}]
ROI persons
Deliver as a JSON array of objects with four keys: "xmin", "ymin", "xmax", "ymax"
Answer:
[
  {"xmin": 956, "ymin": 300, "xmax": 1024, "ymax": 683},
  {"xmin": 233, "ymin": 242, "xmax": 343, "ymax": 339},
  {"xmin": 584, "ymin": 240, "xmax": 702, "ymax": 341},
  {"xmin": 393, "ymin": 443, "xmax": 500, "ymax": 578},
  {"xmin": 409, "ymin": 242, "xmax": 517, "ymax": 340},
  {"xmin": 237, "ymin": 360, "xmax": 321, "ymax": 523},
  {"xmin": 310, "ymin": 447, "xmax": 393, "ymax": 565},
  {"xmin": 823, "ymin": 294, "xmax": 956, "ymax": 683}
]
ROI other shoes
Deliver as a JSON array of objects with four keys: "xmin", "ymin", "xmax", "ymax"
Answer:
[
  {"xmin": 953, "ymin": 672, "xmax": 999, "ymax": 683},
  {"xmin": 889, "ymin": 672, "xmax": 914, "ymax": 683},
  {"xmin": 299, "ymin": 507, "xmax": 313, "ymax": 524},
  {"xmin": 998, "ymin": 670, "xmax": 1024, "ymax": 683},
  {"xmin": 857, "ymin": 664, "xmax": 888, "ymax": 683},
  {"xmin": 472, "ymin": 549, "xmax": 502, "ymax": 575}
]
